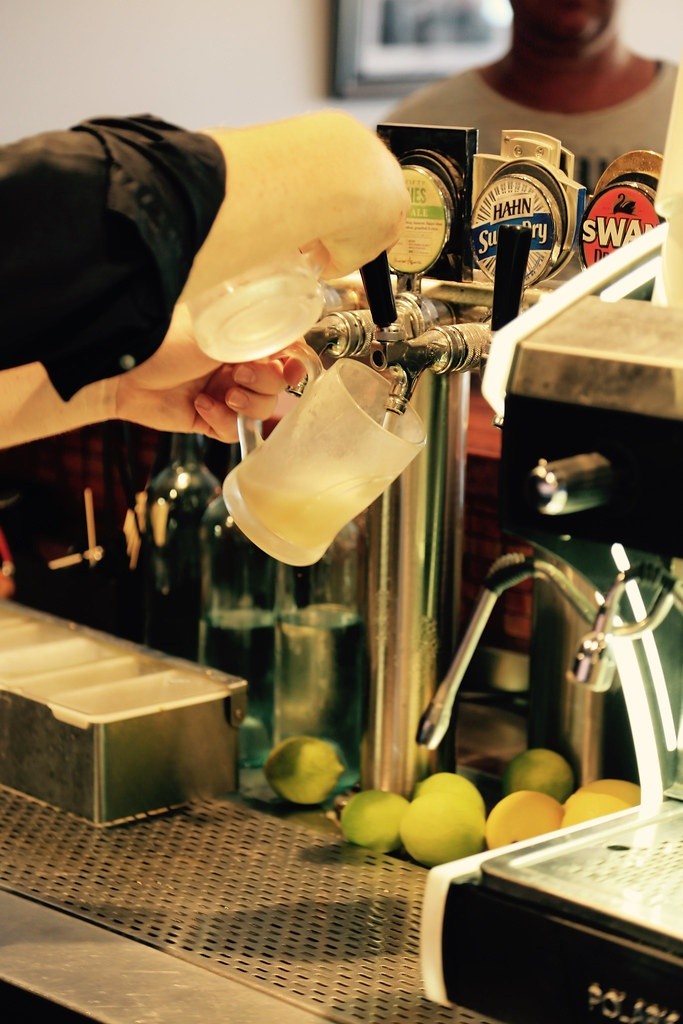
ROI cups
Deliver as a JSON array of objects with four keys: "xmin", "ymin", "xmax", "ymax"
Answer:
[
  {"xmin": 179, "ymin": 240, "xmax": 332, "ymax": 363},
  {"xmin": 221, "ymin": 341, "xmax": 429, "ymax": 568}
]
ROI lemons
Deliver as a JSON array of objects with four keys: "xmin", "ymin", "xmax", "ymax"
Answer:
[{"xmin": 265, "ymin": 736, "xmax": 640, "ymax": 870}]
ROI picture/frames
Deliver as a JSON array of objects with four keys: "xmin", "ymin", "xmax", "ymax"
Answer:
[{"xmin": 335, "ymin": 1, "xmax": 515, "ymax": 101}]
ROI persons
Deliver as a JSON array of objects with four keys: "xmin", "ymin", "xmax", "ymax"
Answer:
[
  {"xmin": 378, "ymin": 0, "xmax": 680, "ymax": 197},
  {"xmin": 0, "ymin": 113, "xmax": 412, "ymax": 450}
]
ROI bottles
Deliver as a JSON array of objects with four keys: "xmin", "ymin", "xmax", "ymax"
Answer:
[{"xmin": 130, "ymin": 427, "xmax": 370, "ymax": 796}]
